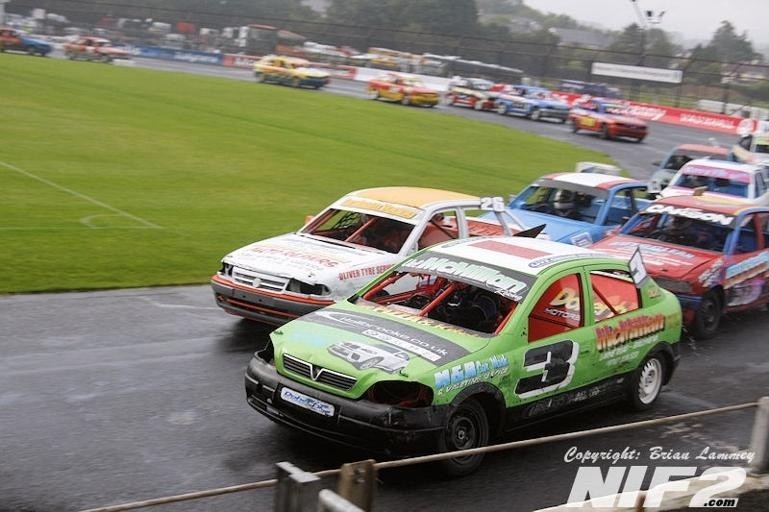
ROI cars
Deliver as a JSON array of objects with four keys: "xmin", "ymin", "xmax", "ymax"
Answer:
[
  {"xmin": 209, "ymin": 133, "xmax": 769, "ymax": 477},
  {"xmin": 0, "ymin": 28, "xmax": 55, "ymax": 58},
  {"xmin": 62, "ymin": 36, "xmax": 129, "ymax": 64},
  {"xmin": 251, "ymin": 42, "xmax": 648, "ymax": 141}
]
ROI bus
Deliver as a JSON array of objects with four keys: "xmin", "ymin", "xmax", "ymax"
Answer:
[{"xmin": 35, "ymin": 8, "xmax": 309, "ymax": 57}]
